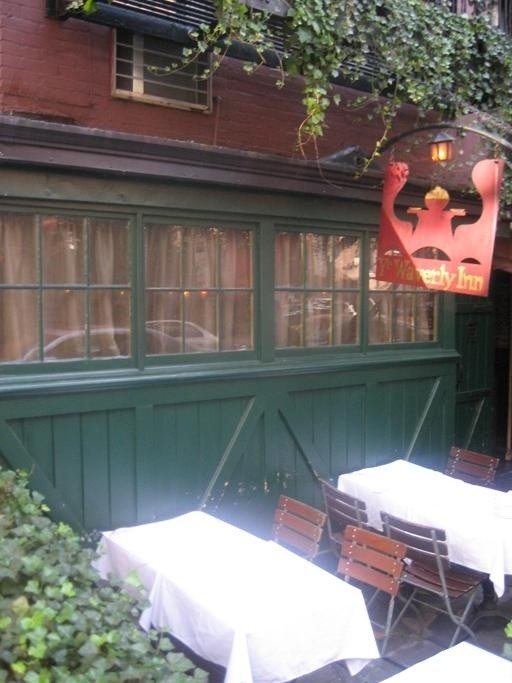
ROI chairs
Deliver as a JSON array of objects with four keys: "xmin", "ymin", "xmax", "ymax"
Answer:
[{"xmin": 269, "ymin": 446, "xmax": 511, "ymax": 651}]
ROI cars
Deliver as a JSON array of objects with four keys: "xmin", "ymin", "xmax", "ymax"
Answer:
[
  {"xmin": 23, "ymin": 319, "xmax": 216, "ymax": 362},
  {"xmin": 283, "ymin": 298, "xmax": 434, "ymax": 347}
]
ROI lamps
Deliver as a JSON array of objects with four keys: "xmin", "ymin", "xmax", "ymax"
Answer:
[{"xmin": 369, "ymin": 120, "xmax": 457, "ymax": 163}]
